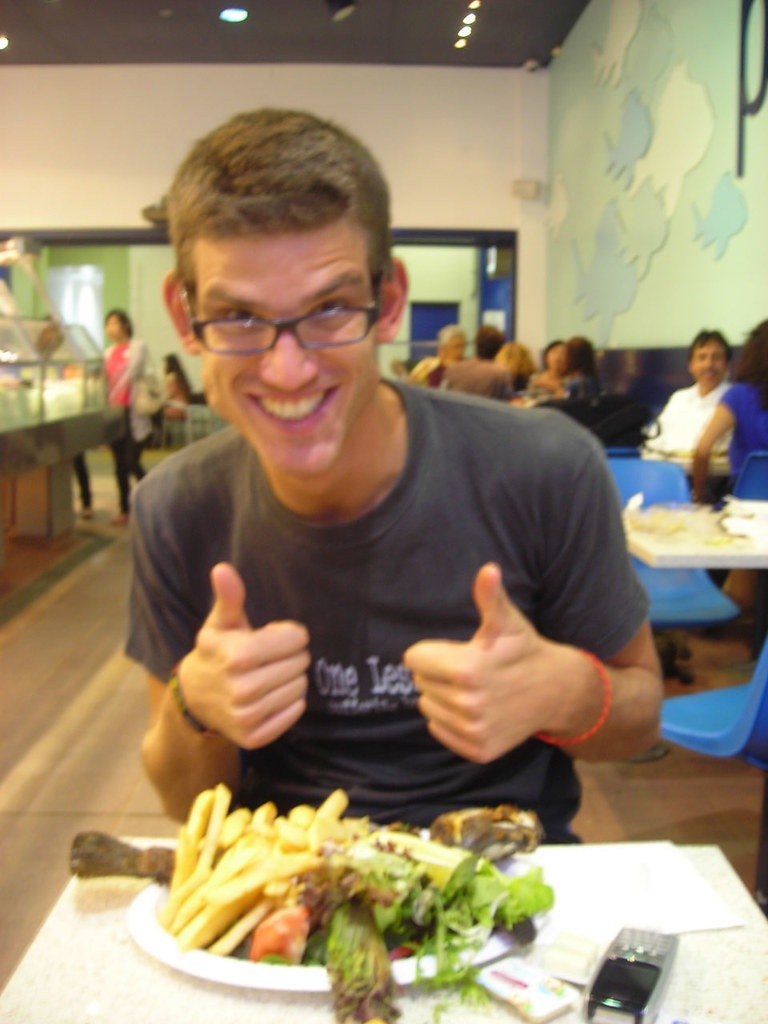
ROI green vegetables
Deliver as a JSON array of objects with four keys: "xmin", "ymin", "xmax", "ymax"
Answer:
[{"xmin": 300, "ymin": 852, "xmax": 554, "ymax": 1003}]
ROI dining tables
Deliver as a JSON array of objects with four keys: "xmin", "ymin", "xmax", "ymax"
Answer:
[{"xmin": 0, "ymin": 833, "xmax": 768, "ymax": 1024}]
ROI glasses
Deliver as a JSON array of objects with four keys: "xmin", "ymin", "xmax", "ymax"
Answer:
[{"xmin": 181, "ymin": 262, "xmax": 383, "ymax": 353}]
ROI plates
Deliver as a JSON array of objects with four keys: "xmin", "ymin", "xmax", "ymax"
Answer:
[{"xmin": 128, "ymin": 842, "xmax": 535, "ymax": 993}]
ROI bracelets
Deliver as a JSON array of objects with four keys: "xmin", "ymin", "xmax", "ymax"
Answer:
[
  {"xmin": 170, "ymin": 663, "xmax": 226, "ymax": 739},
  {"xmin": 530, "ymin": 642, "xmax": 613, "ymax": 749}
]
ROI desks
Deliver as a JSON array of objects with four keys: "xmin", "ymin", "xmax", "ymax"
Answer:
[{"xmin": 624, "ymin": 518, "xmax": 768, "ymax": 675}]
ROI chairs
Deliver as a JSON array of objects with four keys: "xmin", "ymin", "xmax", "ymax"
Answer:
[
  {"xmin": 601, "ymin": 458, "xmax": 737, "ymax": 684},
  {"xmin": 725, "ymin": 449, "xmax": 768, "ymax": 505},
  {"xmin": 650, "ymin": 624, "xmax": 768, "ymax": 905}
]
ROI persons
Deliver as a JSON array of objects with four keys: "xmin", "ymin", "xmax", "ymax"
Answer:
[
  {"xmin": 37, "ymin": 315, "xmax": 93, "ymax": 520},
  {"xmin": 125, "ymin": 110, "xmax": 662, "ymax": 844},
  {"xmin": 408, "ymin": 324, "xmax": 535, "ymax": 402},
  {"xmin": 510, "ymin": 337, "xmax": 601, "ymax": 407},
  {"xmin": 647, "ymin": 318, "xmax": 767, "ymax": 510},
  {"xmin": 103, "ymin": 311, "xmax": 166, "ymax": 526},
  {"xmin": 151, "ymin": 353, "xmax": 191, "ymax": 449}
]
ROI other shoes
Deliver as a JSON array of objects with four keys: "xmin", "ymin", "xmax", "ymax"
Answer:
[
  {"xmin": 83, "ymin": 505, "xmax": 94, "ymax": 519},
  {"xmin": 113, "ymin": 513, "xmax": 131, "ymax": 526}
]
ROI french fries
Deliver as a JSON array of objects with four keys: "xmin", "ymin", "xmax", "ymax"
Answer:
[{"xmin": 166, "ymin": 781, "xmax": 347, "ymax": 955}]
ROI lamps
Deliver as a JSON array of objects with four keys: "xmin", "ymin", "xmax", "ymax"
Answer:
[{"xmin": 325, "ymin": 0, "xmax": 355, "ymax": 22}]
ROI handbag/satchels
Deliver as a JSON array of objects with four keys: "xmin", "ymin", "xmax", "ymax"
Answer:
[{"xmin": 134, "ymin": 371, "xmax": 167, "ymax": 416}]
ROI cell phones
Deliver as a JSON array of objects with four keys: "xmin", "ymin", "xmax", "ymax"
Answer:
[{"xmin": 582, "ymin": 926, "xmax": 680, "ymax": 1024}]
